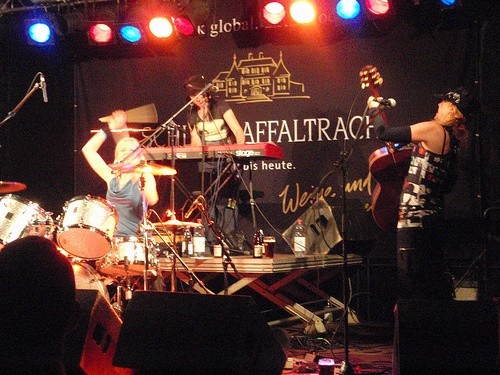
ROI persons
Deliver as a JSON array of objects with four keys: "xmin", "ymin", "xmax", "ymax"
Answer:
[
  {"xmin": 183, "ymin": 75, "xmax": 245, "ymax": 243},
  {"xmin": 367, "ymin": 86, "xmax": 481, "ymax": 299},
  {"xmin": 0, "ymin": 236, "xmax": 77, "ymax": 375},
  {"xmin": 81, "ymin": 110, "xmax": 159, "ymax": 232}
]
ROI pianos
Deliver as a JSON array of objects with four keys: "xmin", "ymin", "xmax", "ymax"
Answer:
[{"xmin": 143, "ymin": 139, "xmax": 283, "ymax": 256}]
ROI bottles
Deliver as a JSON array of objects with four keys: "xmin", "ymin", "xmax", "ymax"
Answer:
[
  {"xmin": 191, "ymin": 219, "xmax": 205, "ymax": 258},
  {"xmin": 293, "ymin": 218, "xmax": 307, "ymax": 257},
  {"xmin": 213, "ymin": 231, "xmax": 222, "ymax": 258},
  {"xmin": 253, "ymin": 230, "xmax": 262, "ymax": 258},
  {"xmin": 318, "ymin": 358, "xmax": 335, "ymax": 375},
  {"xmin": 260, "ymin": 229, "xmax": 266, "ymax": 255},
  {"xmin": 181, "ymin": 226, "xmax": 194, "ymax": 258},
  {"xmin": 322, "ymin": 299, "xmax": 333, "ymax": 325}
]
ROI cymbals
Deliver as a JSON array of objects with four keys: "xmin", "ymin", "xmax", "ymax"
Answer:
[
  {"xmin": 152, "ymin": 220, "xmax": 202, "ymax": 231},
  {"xmin": 0, "ymin": 181, "xmax": 27, "ymax": 193},
  {"xmin": 108, "ymin": 162, "xmax": 177, "ymax": 176}
]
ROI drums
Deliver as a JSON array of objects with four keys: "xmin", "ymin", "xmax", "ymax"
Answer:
[
  {"xmin": 0, "ymin": 194, "xmax": 57, "ymax": 247},
  {"xmin": 95, "ymin": 236, "xmax": 159, "ymax": 278},
  {"xmin": 71, "ymin": 261, "xmax": 110, "ymax": 302},
  {"xmin": 56, "ymin": 195, "xmax": 118, "ymax": 260}
]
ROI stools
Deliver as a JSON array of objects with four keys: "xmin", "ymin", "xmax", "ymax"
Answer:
[{"xmin": 226, "ymin": 190, "xmax": 264, "ymax": 255}]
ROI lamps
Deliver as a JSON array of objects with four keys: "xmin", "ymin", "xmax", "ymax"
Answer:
[
  {"xmin": 393, "ymin": 300, "xmax": 500, "ymax": 375},
  {"xmin": 113, "ymin": 291, "xmax": 286, "ymax": 375},
  {"xmin": 23, "ymin": 0, "xmax": 463, "ymax": 46},
  {"xmin": 62, "ymin": 289, "xmax": 134, "ymax": 375}
]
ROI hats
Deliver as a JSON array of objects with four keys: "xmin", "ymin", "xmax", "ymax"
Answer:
[
  {"xmin": 434, "ymin": 86, "xmax": 481, "ymax": 123},
  {"xmin": 182, "ymin": 75, "xmax": 211, "ymax": 92}
]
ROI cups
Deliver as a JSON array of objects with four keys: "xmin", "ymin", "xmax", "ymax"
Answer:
[{"xmin": 263, "ymin": 237, "xmax": 276, "ymax": 260}]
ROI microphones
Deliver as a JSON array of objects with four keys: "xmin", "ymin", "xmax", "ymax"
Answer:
[
  {"xmin": 202, "ymin": 86, "xmax": 219, "ymax": 94},
  {"xmin": 40, "ymin": 74, "xmax": 49, "ymax": 103},
  {"xmin": 373, "ymin": 98, "xmax": 397, "ymax": 108}
]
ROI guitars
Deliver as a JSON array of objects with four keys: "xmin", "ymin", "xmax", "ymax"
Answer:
[{"xmin": 359, "ymin": 64, "xmax": 414, "ymax": 233}]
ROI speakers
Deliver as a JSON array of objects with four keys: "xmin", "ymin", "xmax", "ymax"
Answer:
[
  {"xmin": 393, "ymin": 299, "xmax": 499, "ymax": 375},
  {"xmin": 112, "ymin": 289, "xmax": 287, "ymax": 375},
  {"xmin": 62, "ymin": 289, "xmax": 133, "ymax": 375}
]
